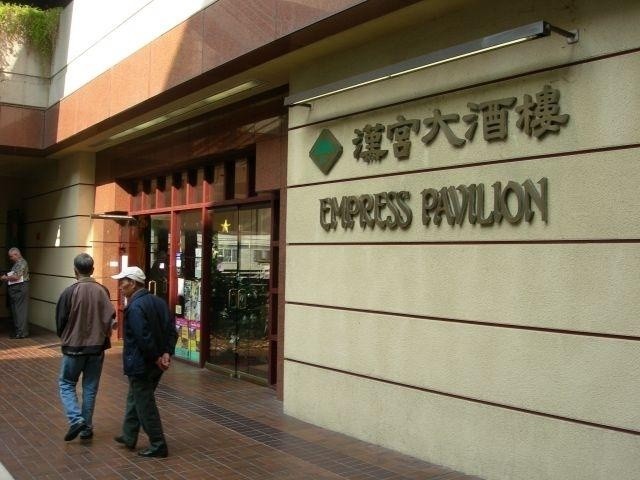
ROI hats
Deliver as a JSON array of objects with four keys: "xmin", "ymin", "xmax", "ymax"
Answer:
[{"xmin": 109, "ymin": 265, "xmax": 146, "ymax": 284}]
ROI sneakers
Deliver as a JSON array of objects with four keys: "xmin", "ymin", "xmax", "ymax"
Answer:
[
  {"xmin": 111, "ymin": 434, "xmax": 138, "ymax": 450},
  {"xmin": 63, "ymin": 421, "xmax": 94, "ymax": 442},
  {"xmin": 136, "ymin": 446, "xmax": 169, "ymax": 458},
  {"xmin": 9, "ymin": 330, "xmax": 29, "ymax": 339}
]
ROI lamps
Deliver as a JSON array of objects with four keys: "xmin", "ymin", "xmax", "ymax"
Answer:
[{"xmin": 284, "ymin": 19, "xmax": 579, "ymax": 110}]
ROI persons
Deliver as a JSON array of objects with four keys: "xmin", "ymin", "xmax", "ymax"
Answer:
[
  {"xmin": 1, "ymin": 247, "xmax": 31, "ymax": 339},
  {"xmin": 54, "ymin": 252, "xmax": 118, "ymax": 442},
  {"xmin": 110, "ymin": 265, "xmax": 177, "ymax": 457}
]
